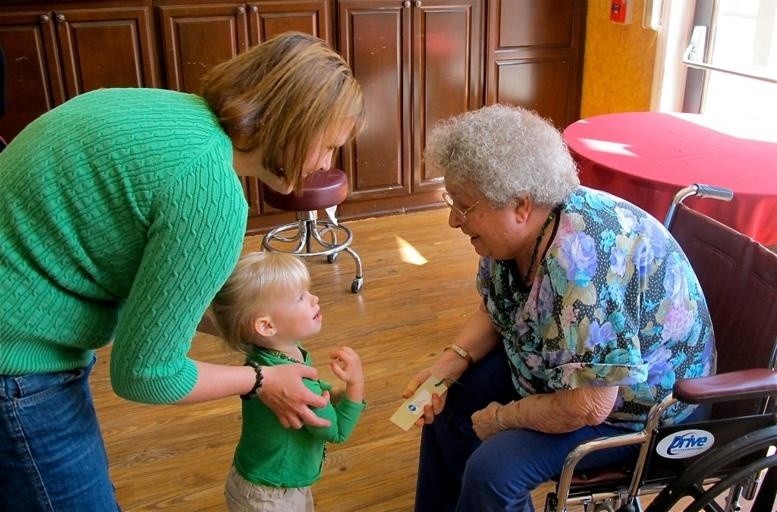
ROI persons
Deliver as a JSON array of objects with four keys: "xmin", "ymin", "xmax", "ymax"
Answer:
[
  {"xmin": 2, "ymin": 29, "xmax": 367, "ymax": 512},
  {"xmin": 206, "ymin": 250, "xmax": 368, "ymax": 512},
  {"xmin": 401, "ymin": 103, "xmax": 719, "ymax": 511}
]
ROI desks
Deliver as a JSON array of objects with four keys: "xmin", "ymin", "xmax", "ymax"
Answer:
[{"xmin": 559, "ymin": 112, "xmax": 777, "ymax": 254}]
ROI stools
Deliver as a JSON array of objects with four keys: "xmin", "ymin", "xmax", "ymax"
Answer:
[{"xmin": 259, "ymin": 168, "xmax": 365, "ymax": 295}]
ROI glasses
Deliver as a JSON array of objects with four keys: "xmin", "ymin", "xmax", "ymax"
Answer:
[{"xmin": 440, "ymin": 190, "xmax": 487, "ymax": 226}]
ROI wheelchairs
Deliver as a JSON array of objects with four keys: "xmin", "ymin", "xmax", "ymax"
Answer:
[{"xmin": 544, "ymin": 183, "xmax": 777, "ymax": 512}]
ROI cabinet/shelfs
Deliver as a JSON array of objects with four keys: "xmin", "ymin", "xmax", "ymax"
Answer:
[{"xmin": 0, "ymin": 0, "xmax": 589, "ymax": 237}]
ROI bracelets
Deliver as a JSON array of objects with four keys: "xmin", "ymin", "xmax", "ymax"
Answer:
[
  {"xmin": 443, "ymin": 342, "xmax": 476, "ymax": 368},
  {"xmin": 240, "ymin": 361, "xmax": 266, "ymax": 402}
]
